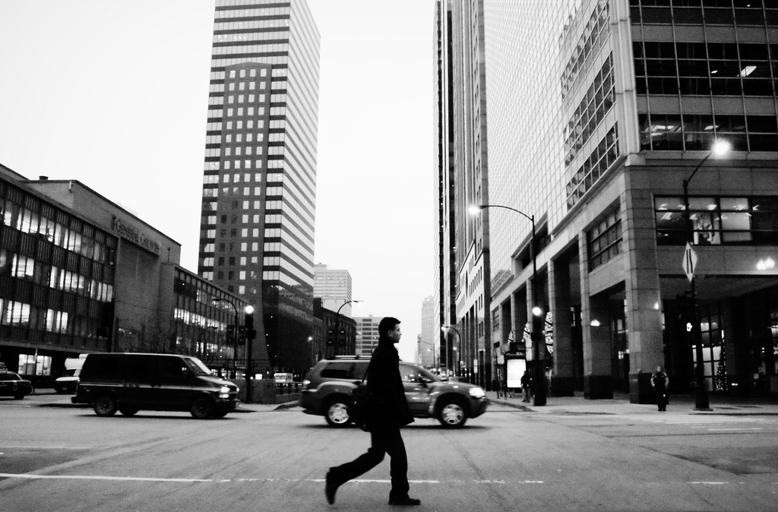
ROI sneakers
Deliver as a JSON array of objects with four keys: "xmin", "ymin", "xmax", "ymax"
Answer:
[
  {"xmin": 389, "ymin": 495, "xmax": 420, "ymax": 506},
  {"xmin": 326, "ymin": 469, "xmax": 339, "ymax": 504}
]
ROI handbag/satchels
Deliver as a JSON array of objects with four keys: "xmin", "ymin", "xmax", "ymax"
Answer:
[{"xmin": 347, "ymin": 386, "xmax": 371, "ymax": 432}]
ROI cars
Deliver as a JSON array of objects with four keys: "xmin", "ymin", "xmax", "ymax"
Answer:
[
  {"xmin": 210, "ymin": 365, "xmax": 299, "ymax": 386},
  {"xmin": 0, "ymin": 363, "xmax": 33, "ymax": 400},
  {"xmin": 425, "ymin": 366, "xmax": 454, "ymax": 381}
]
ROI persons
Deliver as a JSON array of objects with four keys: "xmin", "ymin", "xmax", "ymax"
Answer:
[
  {"xmin": 323, "ymin": 315, "xmax": 422, "ymax": 506},
  {"xmin": 211, "ymin": 361, "xmax": 672, "ymax": 411}
]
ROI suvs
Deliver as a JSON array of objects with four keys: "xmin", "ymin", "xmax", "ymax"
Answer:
[{"xmin": 297, "ymin": 353, "xmax": 491, "ymax": 430}]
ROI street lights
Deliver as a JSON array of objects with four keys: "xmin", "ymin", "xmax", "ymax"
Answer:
[
  {"xmin": 468, "ymin": 202, "xmax": 541, "ymax": 406},
  {"xmin": 427, "ymin": 347, "xmax": 436, "ymax": 366},
  {"xmin": 680, "ymin": 135, "xmax": 732, "ymax": 412},
  {"xmin": 243, "ymin": 305, "xmax": 258, "ymax": 380},
  {"xmin": 326, "ymin": 300, "xmax": 360, "ymax": 354},
  {"xmin": 209, "ymin": 297, "xmax": 240, "ymax": 366},
  {"xmin": 440, "ymin": 324, "xmax": 462, "ymax": 376}
]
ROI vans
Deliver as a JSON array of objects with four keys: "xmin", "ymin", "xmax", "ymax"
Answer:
[{"xmin": 69, "ymin": 350, "xmax": 242, "ymax": 420}]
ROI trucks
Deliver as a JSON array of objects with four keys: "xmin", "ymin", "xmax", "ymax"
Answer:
[{"xmin": 54, "ymin": 353, "xmax": 88, "ymax": 394}]
